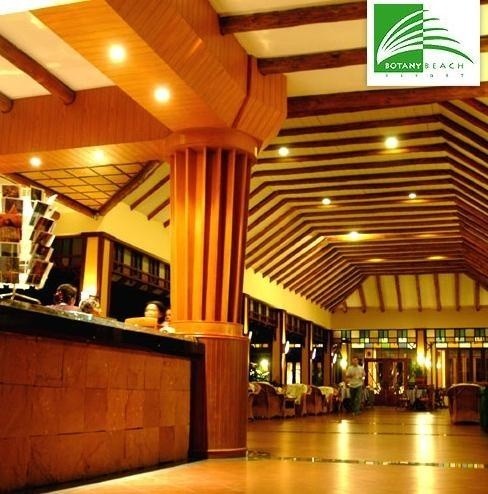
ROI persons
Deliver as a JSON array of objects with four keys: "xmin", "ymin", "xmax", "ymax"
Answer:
[
  {"xmin": 49, "ymin": 283, "xmax": 104, "ymax": 317},
  {"xmin": 144, "ymin": 300, "xmax": 176, "ymax": 333},
  {"xmin": 344, "ymin": 356, "xmax": 366, "ymax": 416}
]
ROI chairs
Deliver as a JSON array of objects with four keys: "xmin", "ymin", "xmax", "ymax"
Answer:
[
  {"xmin": 416, "ymin": 384, "xmax": 481, "ymax": 423},
  {"xmin": 246, "ymin": 380, "xmax": 346, "ymax": 422}
]
